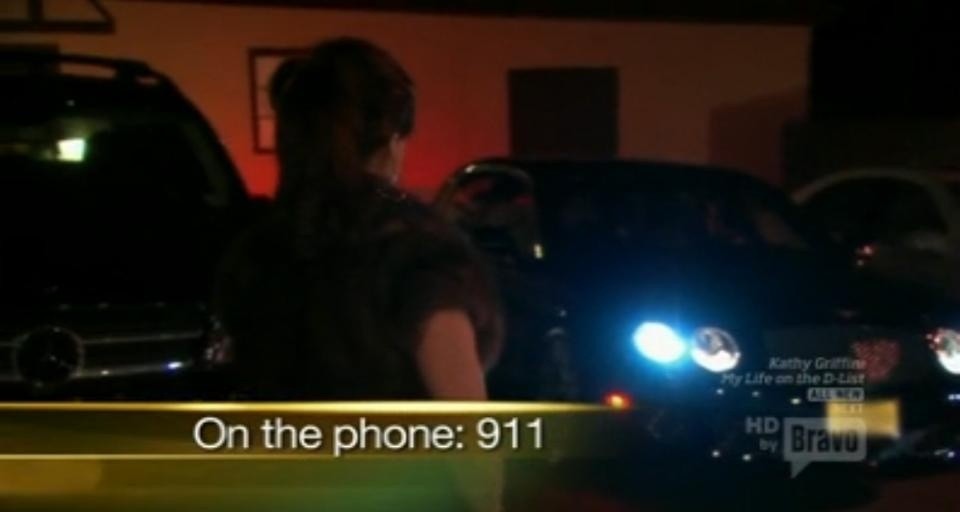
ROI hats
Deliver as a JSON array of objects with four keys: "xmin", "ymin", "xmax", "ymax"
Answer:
[
  {"xmin": 426, "ymin": 152, "xmax": 951, "ymax": 509},
  {"xmin": 777, "ymin": 159, "xmax": 951, "ymax": 277},
  {"xmin": 10, "ymin": 42, "xmax": 265, "ymax": 405}
]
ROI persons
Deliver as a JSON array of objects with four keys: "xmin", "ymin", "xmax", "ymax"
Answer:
[{"xmin": 218, "ymin": 36, "xmax": 498, "ymax": 401}]
